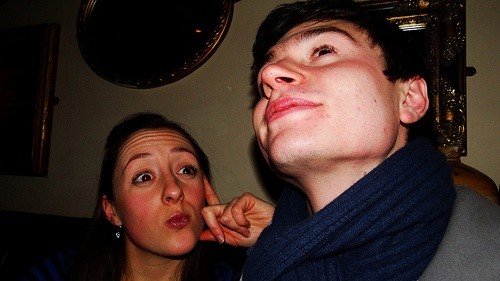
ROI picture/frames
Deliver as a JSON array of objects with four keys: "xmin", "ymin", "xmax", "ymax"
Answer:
[
  {"xmin": 1, "ymin": 21, "xmax": 61, "ymax": 177},
  {"xmin": 351, "ymin": 0, "xmax": 467, "ymax": 158}
]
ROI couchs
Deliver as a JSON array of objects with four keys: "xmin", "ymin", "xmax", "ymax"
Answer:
[{"xmin": 0, "ymin": 208, "xmax": 248, "ymax": 281}]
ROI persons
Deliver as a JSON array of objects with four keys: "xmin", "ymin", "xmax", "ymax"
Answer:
[
  {"xmin": 28, "ymin": 112, "xmax": 277, "ymax": 281},
  {"xmin": 241, "ymin": 1, "xmax": 500, "ymax": 281}
]
